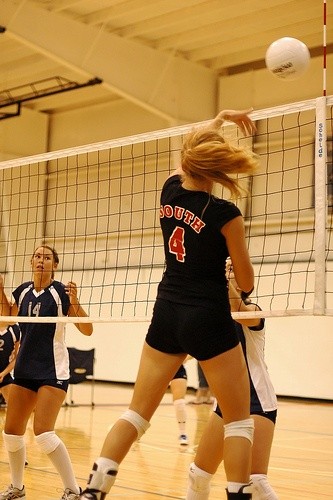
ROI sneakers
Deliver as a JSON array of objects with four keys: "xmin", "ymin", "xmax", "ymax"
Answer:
[
  {"xmin": 180, "ymin": 434, "xmax": 188, "ymax": 445},
  {"xmin": 59, "ymin": 487, "xmax": 83, "ymax": 500},
  {"xmin": 189, "ymin": 398, "xmax": 214, "ymax": 404},
  {"xmin": 0, "ymin": 484, "xmax": 26, "ymax": 500}
]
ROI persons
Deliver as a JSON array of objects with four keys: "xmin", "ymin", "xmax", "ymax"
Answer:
[
  {"xmin": 88, "ymin": 108, "xmax": 262, "ymax": 500},
  {"xmin": 166, "ymin": 354, "xmax": 190, "ymax": 446},
  {"xmin": 181, "ymin": 258, "xmax": 279, "ymax": 500},
  {"xmin": 0, "ymin": 244, "xmax": 93, "ymax": 500},
  {"xmin": 1, "ymin": 318, "xmax": 27, "ymax": 468}
]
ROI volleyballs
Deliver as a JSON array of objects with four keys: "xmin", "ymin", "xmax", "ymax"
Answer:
[{"xmin": 264, "ymin": 37, "xmax": 310, "ymax": 83}]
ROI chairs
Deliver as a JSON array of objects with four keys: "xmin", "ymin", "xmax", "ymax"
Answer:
[{"xmin": 65, "ymin": 346, "xmax": 97, "ymax": 408}]
